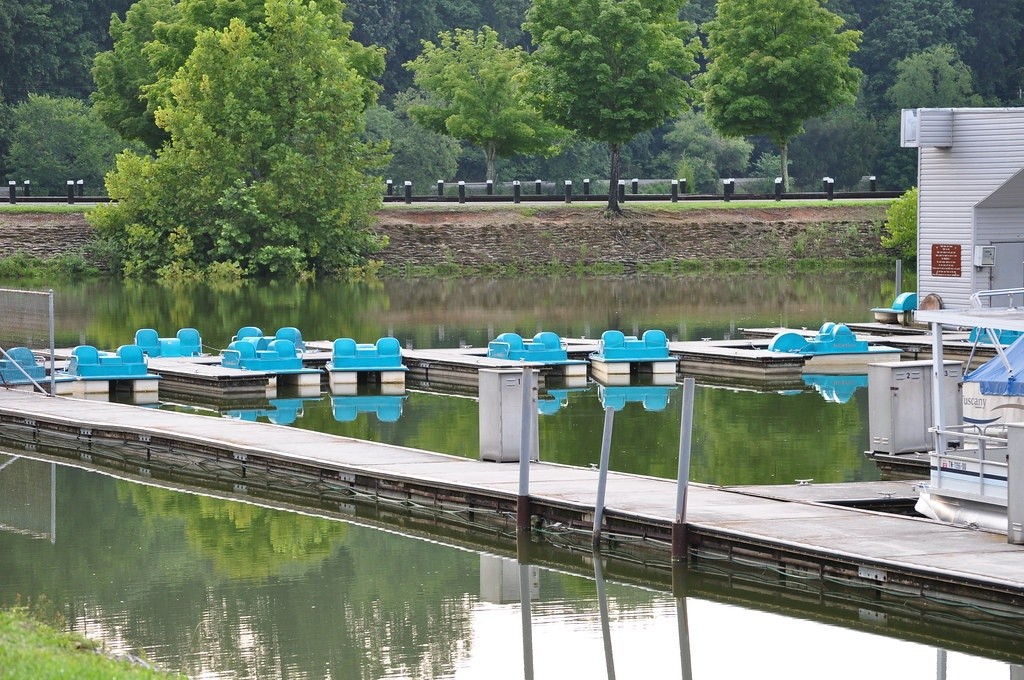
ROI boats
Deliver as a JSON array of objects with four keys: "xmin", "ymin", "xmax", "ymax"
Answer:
[
  {"xmin": 219, "ymin": 339, "xmax": 325, "ymax": 387},
  {"xmin": 58, "ymin": 345, "xmax": 163, "ymax": 394},
  {"xmin": 488, "ymin": 332, "xmax": 590, "ymax": 377},
  {"xmin": 232, "ymin": 327, "xmax": 306, "ymax": 353},
  {"xmin": 766, "ymin": 322, "xmax": 904, "ymax": 365},
  {"xmin": 588, "ymin": 330, "xmax": 680, "ymax": 373},
  {"xmin": 134, "ymin": 328, "xmax": 203, "ymax": 358},
  {"xmin": 0, "ymin": 346, "xmax": 85, "ymax": 396},
  {"xmin": 871, "ymin": 292, "xmax": 917, "ymax": 324},
  {"xmin": 325, "ymin": 338, "xmax": 409, "ymax": 383}
]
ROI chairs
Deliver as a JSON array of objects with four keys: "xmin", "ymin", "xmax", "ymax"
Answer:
[
  {"xmin": 217, "ymin": 396, "xmax": 406, "ymax": 426},
  {"xmin": 1, "ymin": 326, "xmax": 406, "ymax": 375},
  {"xmin": 770, "ymin": 318, "xmax": 869, "ymax": 356},
  {"xmin": 801, "ymin": 375, "xmax": 868, "ymax": 404},
  {"xmin": 538, "ymin": 385, "xmax": 674, "ymax": 414},
  {"xmin": 491, "ymin": 328, "xmax": 679, "ymax": 365}
]
